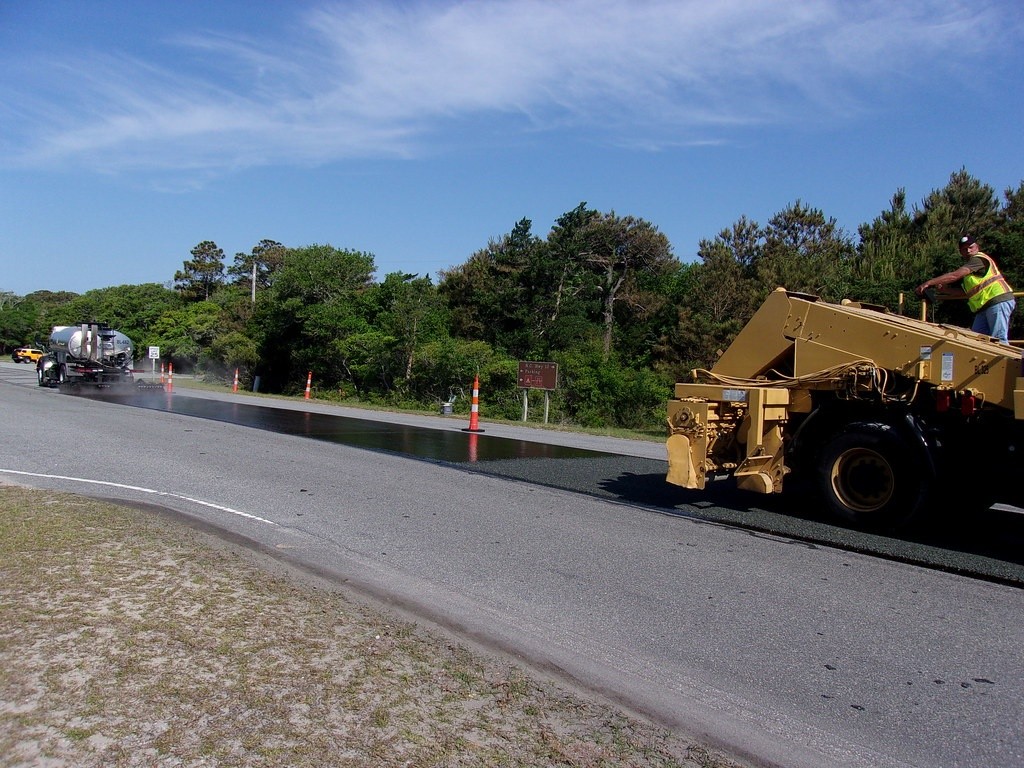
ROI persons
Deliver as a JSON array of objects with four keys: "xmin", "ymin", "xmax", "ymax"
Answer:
[{"xmin": 913, "ymin": 235, "xmax": 1017, "ymax": 346}]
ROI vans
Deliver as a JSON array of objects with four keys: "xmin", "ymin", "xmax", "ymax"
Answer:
[{"xmin": 12, "ymin": 348, "xmax": 45, "ymax": 364}]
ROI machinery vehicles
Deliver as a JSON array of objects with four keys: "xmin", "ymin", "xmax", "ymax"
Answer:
[
  {"xmin": 36, "ymin": 321, "xmax": 166, "ymax": 396},
  {"xmin": 664, "ymin": 286, "xmax": 1024, "ymax": 537}
]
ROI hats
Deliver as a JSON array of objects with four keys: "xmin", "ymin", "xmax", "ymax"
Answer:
[{"xmin": 958, "ymin": 235, "xmax": 976, "ymax": 249}]
